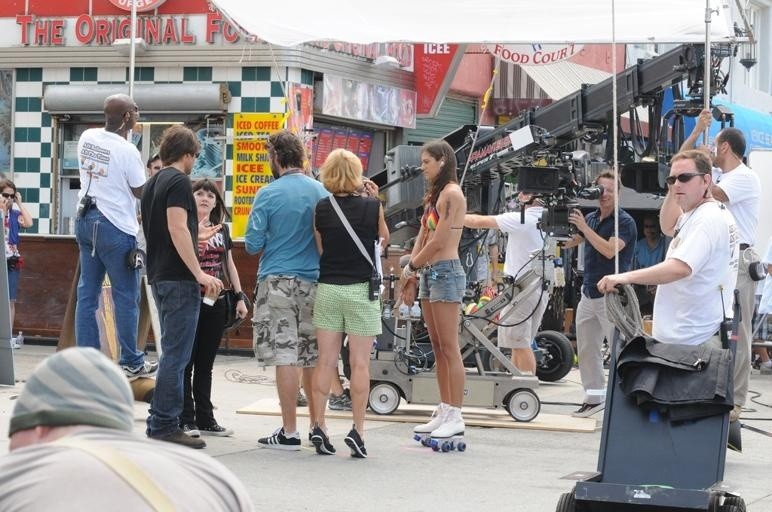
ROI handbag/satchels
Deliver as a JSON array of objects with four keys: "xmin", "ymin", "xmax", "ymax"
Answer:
[
  {"xmin": 373, "ymin": 237, "xmax": 384, "ymax": 284},
  {"xmin": 214, "ymin": 290, "xmax": 251, "ymax": 331}
]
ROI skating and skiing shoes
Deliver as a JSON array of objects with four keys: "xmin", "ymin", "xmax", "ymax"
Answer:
[
  {"xmin": 430, "ymin": 406, "xmax": 466, "ymax": 452},
  {"xmin": 413, "ymin": 402, "xmax": 449, "ymax": 447}
]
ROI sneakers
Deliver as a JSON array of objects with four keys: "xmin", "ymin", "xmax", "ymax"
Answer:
[
  {"xmin": 147, "ymin": 421, "xmax": 234, "ymax": 449},
  {"xmin": 572, "ymin": 399, "xmax": 605, "ymax": 418},
  {"xmin": 258, "ymin": 423, "xmax": 367, "ymax": 458},
  {"xmin": 296, "ymin": 391, "xmax": 307, "ymax": 407},
  {"xmin": 120, "ymin": 360, "xmax": 159, "ymax": 381},
  {"xmin": 329, "ymin": 392, "xmax": 354, "ymax": 410}
]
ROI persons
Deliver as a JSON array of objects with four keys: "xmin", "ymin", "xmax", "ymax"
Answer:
[
  {"xmin": 397, "ymin": 140, "xmax": 467, "ymax": 440},
  {"xmin": 179, "ymin": 177, "xmax": 249, "ymax": 437},
  {"xmin": 754, "ymin": 243, "xmax": 772, "ymax": 374},
  {"xmin": 135, "ymin": 151, "xmax": 163, "ymax": 253},
  {"xmin": 676, "ymin": 109, "xmax": 761, "ymax": 420},
  {"xmin": 142, "ymin": 122, "xmax": 224, "ymax": 448},
  {"xmin": 75, "ymin": 94, "xmax": 159, "ymax": 383},
  {"xmin": 464, "ymin": 191, "xmax": 555, "ymax": 377},
  {"xmin": 0, "ymin": 346, "xmax": 249, "ymax": 512},
  {"xmin": 0, "ymin": 177, "xmax": 33, "ymax": 349},
  {"xmin": 560, "ymin": 172, "xmax": 637, "ymax": 417},
  {"xmin": 595, "ymin": 149, "xmax": 738, "ymax": 346},
  {"xmin": 243, "ymin": 130, "xmax": 392, "ymax": 457},
  {"xmin": 630, "ymin": 214, "xmax": 665, "ymax": 306}
]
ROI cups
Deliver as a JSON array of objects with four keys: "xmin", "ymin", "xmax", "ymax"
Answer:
[{"xmin": 203, "ymin": 286, "xmax": 221, "ymax": 307}]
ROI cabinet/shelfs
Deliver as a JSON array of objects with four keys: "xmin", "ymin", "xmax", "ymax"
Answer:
[{"xmin": 13, "ymin": 239, "xmax": 402, "ymax": 356}]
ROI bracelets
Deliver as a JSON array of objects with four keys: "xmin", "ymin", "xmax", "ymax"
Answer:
[
  {"xmin": 403, "ymin": 264, "xmax": 412, "ymax": 280},
  {"xmin": 409, "ymin": 261, "xmax": 416, "ymax": 272},
  {"xmin": 235, "ymin": 293, "xmax": 244, "ymax": 300}
]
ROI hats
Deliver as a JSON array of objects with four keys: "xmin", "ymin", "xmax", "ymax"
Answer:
[{"xmin": 8, "ymin": 345, "xmax": 135, "ymax": 437}]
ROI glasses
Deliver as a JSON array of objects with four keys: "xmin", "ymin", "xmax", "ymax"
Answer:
[
  {"xmin": 1, "ymin": 193, "xmax": 15, "ymax": 198},
  {"xmin": 665, "ymin": 172, "xmax": 705, "ymax": 186}
]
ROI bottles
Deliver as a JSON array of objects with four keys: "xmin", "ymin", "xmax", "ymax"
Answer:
[{"xmin": 409, "ymin": 300, "xmax": 421, "ymax": 321}]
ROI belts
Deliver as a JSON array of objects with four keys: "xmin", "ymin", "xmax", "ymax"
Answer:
[{"xmin": 740, "ymin": 244, "xmax": 750, "ymax": 250}]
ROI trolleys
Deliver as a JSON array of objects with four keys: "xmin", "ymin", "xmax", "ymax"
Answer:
[
  {"xmin": 335, "ymin": 234, "xmax": 579, "ymax": 425},
  {"xmin": 551, "ymin": 285, "xmax": 747, "ymax": 512}
]
